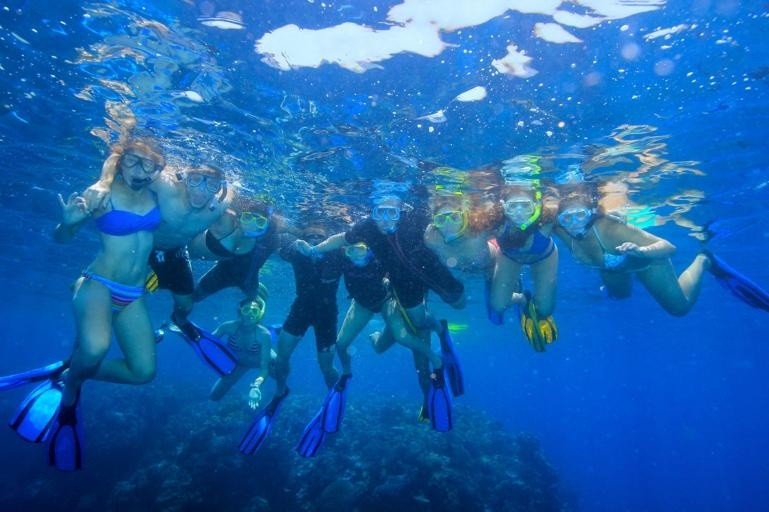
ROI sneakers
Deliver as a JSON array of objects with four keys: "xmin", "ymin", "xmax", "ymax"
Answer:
[
  {"xmin": 267, "ymin": 387, "xmax": 290, "ymax": 414},
  {"xmin": 698, "ymin": 249, "xmax": 731, "ymax": 282},
  {"xmin": 170, "ymin": 312, "xmax": 200, "ymax": 343},
  {"xmin": 424, "ymin": 389, "xmax": 431, "ymax": 409},
  {"xmin": 57, "ymin": 364, "xmax": 82, "ymax": 425},
  {"xmin": 333, "ymin": 372, "xmax": 353, "ymax": 395},
  {"xmin": 522, "ymin": 289, "xmax": 547, "ymax": 322},
  {"xmin": 431, "ymin": 358, "xmax": 445, "ymax": 390},
  {"xmin": 436, "ymin": 317, "xmax": 453, "ymax": 355}
]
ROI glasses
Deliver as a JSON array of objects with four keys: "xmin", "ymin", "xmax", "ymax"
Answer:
[
  {"xmin": 343, "ymin": 244, "xmax": 370, "ymax": 258},
  {"xmin": 240, "ymin": 304, "xmax": 262, "ymax": 319},
  {"xmin": 185, "ymin": 170, "xmax": 223, "ymax": 195},
  {"xmin": 557, "ymin": 207, "xmax": 592, "ymax": 228},
  {"xmin": 240, "ymin": 210, "xmax": 269, "ymax": 229},
  {"xmin": 119, "ymin": 151, "xmax": 158, "ymax": 174},
  {"xmin": 372, "ymin": 205, "xmax": 403, "ymax": 222},
  {"xmin": 502, "ymin": 199, "xmax": 537, "ymax": 216},
  {"xmin": 433, "ymin": 209, "xmax": 465, "ymax": 229}
]
